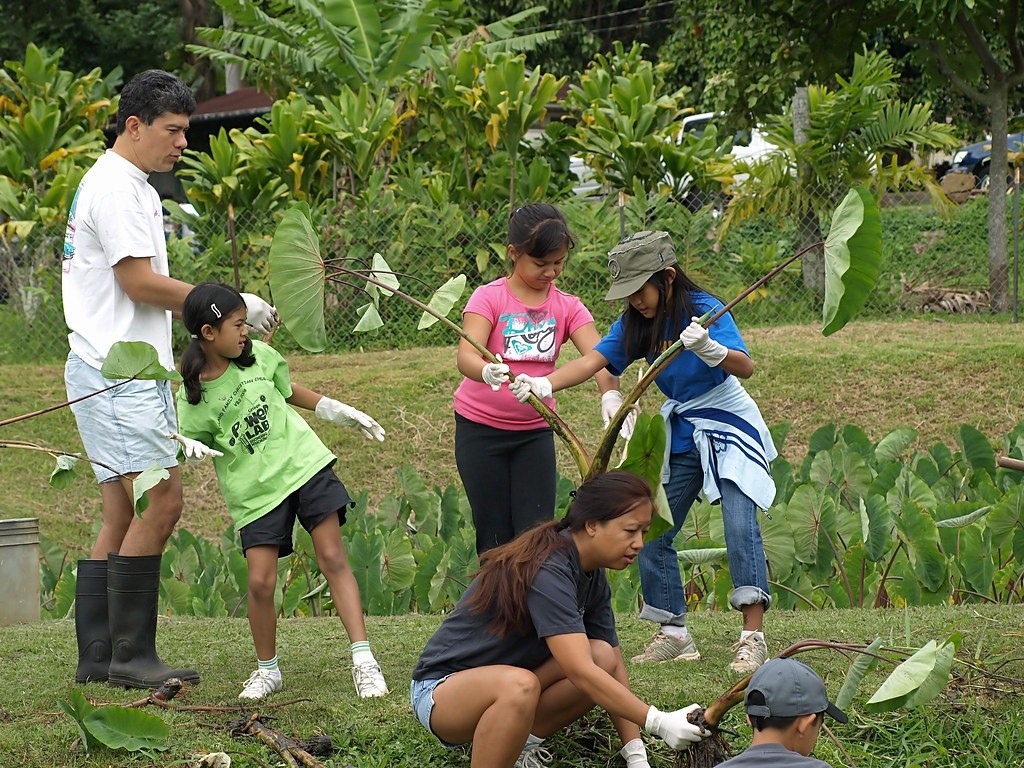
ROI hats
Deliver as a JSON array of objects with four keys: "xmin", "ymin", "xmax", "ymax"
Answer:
[
  {"xmin": 605, "ymin": 230, "xmax": 677, "ymax": 301},
  {"xmin": 744, "ymin": 656, "xmax": 848, "ymax": 724}
]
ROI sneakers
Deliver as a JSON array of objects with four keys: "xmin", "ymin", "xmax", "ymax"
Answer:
[
  {"xmin": 630, "ymin": 630, "xmax": 701, "ymax": 667},
  {"xmin": 239, "ymin": 669, "xmax": 283, "ymax": 700},
  {"xmin": 351, "ymin": 660, "xmax": 389, "ymax": 697},
  {"xmin": 514, "ymin": 745, "xmax": 552, "ymax": 768},
  {"xmin": 727, "ymin": 630, "xmax": 770, "ymax": 673}
]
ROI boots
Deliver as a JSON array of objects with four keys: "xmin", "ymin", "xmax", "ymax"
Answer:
[
  {"xmin": 74, "ymin": 560, "xmax": 112, "ymax": 684},
  {"xmin": 107, "ymin": 551, "xmax": 201, "ymax": 688}
]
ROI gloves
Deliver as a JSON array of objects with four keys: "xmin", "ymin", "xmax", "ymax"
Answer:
[
  {"xmin": 601, "ymin": 390, "xmax": 638, "ymax": 438},
  {"xmin": 509, "ymin": 374, "xmax": 554, "ymax": 404},
  {"xmin": 482, "ymin": 353, "xmax": 512, "ymax": 392},
  {"xmin": 645, "ymin": 703, "xmax": 712, "ymax": 750},
  {"xmin": 315, "ymin": 396, "xmax": 386, "ymax": 442},
  {"xmin": 679, "ymin": 317, "xmax": 728, "ymax": 368},
  {"xmin": 621, "ymin": 739, "xmax": 651, "ymax": 768},
  {"xmin": 171, "ymin": 433, "xmax": 224, "ymax": 459},
  {"xmin": 239, "ymin": 293, "xmax": 280, "ymax": 335}
]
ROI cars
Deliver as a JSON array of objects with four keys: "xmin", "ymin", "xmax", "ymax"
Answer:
[
  {"xmin": 939, "ymin": 130, "xmax": 1024, "ymax": 196},
  {"xmin": 568, "ymin": 111, "xmax": 883, "ymax": 214}
]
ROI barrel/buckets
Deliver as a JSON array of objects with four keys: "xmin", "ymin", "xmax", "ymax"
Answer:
[{"xmin": 0, "ymin": 518, "xmax": 40, "ymax": 626}]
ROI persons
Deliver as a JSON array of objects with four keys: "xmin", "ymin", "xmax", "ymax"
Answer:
[
  {"xmin": 509, "ymin": 230, "xmax": 777, "ymax": 675},
  {"xmin": 454, "ymin": 203, "xmax": 633, "ymax": 570},
  {"xmin": 714, "ymin": 658, "xmax": 848, "ymax": 768},
  {"xmin": 173, "ymin": 282, "xmax": 387, "ymax": 700},
  {"xmin": 409, "ymin": 470, "xmax": 710, "ymax": 768},
  {"xmin": 62, "ymin": 68, "xmax": 276, "ymax": 691}
]
